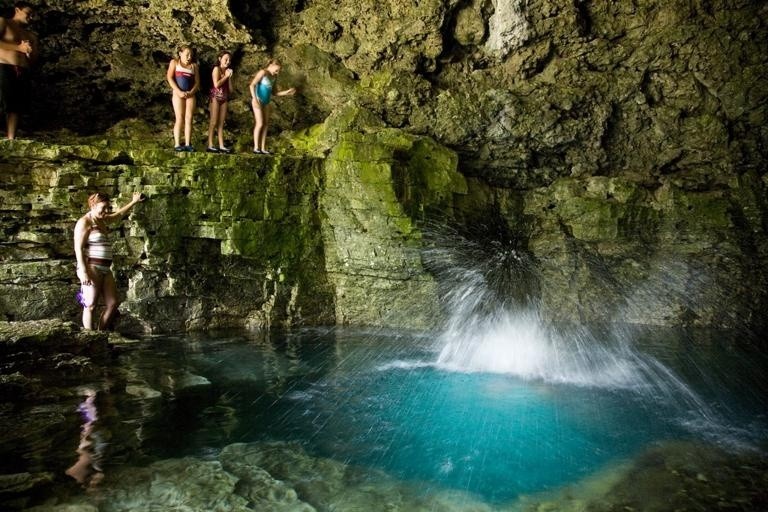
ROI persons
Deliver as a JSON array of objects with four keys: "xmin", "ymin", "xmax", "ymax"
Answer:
[
  {"xmin": 249, "ymin": 57, "xmax": 296, "ymax": 154},
  {"xmin": 0, "ymin": 1, "xmax": 39, "ymax": 138},
  {"xmin": 65, "ymin": 390, "xmax": 117, "ymax": 488},
  {"xmin": 74, "ymin": 190, "xmax": 144, "ymax": 330},
  {"xmin": 206, "ymin": 52, "xmax": 234, "ymax": 153},
  {"xmin": 167, "ymin": 44, "xmax": 200, "ymax": 152}
]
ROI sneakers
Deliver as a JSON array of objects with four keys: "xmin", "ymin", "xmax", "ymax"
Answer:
[
  {"xmin": 185, "ymin": 146, "xmax": 195, "ymax": 152},
  {"xmin": 174, "ymin": 146, "xmax": 184, "ymax": 151},
  {"xmin": 253, "ymin": 148, "xmax": 260, "ymax": 153},
  {"xmin": 219, "ymin": 147, "xmax": 232, "ymax": 153},
  {"xmin": 207, "ymin": 147, "xmax": 219, "ymax": 153},
  {"xmin": 263, "ymin": 150, "xmax": 270, "ymax": 154}
]
